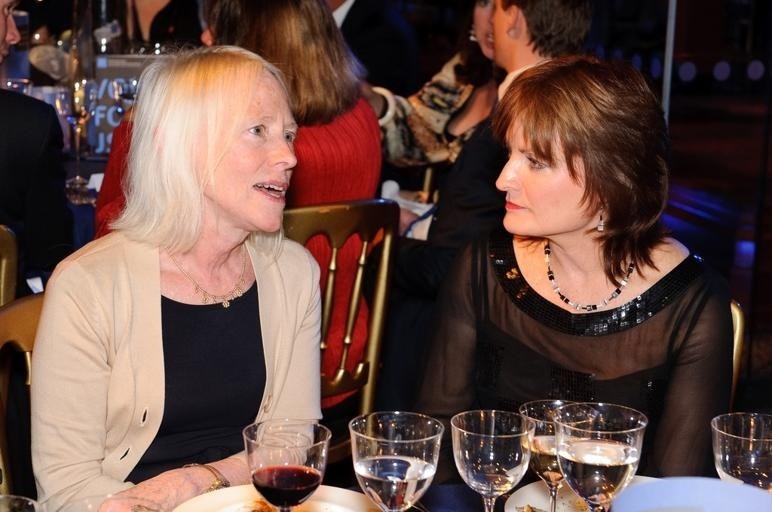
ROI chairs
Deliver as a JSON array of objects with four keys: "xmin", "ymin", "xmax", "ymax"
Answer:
[
  {"xmin": 0, "ymin": 292, "xmax": 44, "ymax": 512},
  {"xmin": 731, "ymin": 296, "xmax": 745, "ymax": 413},
  {"xmin": 283, "ymin": 199, "xmax": 400, "ymax": 471},
  {"xmin": 0, "ymin": 224, "xmax": 19, "ymax": 308}
]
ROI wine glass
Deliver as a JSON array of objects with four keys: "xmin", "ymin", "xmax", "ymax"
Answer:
[
  {"xmin": 0, "ymin": 78, "xmax": 31, "ymax": 98},
  {"xmin": 519, "ymin": 399, "xmax": 580, "ymax": 512},
  {"xmin": 711, "ymin": 412, "xmax": 772, "ymax": 494},
  {"xmin": 113, "ymin": 79, "xmax": 138, "ymax": 121},
  {"xmin": 450, "ymin": 409, "xmax": 536, "ymax": 512},
  {"xmin": 551, "ymin": 403, "xmax": 648, "ymax": 512},
  {"xmin": 349, "ymin": 412, "xmax": 446, "ymax": 512},
  {"xmin": 55, "ymin": 79, "xmax": 97, "ymax": 192},
  {"xmin": 240, "ymin": 420, "xmax": 332, "ymax": 512}
]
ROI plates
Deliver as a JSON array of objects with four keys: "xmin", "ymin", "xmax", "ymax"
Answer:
[
  {"xmin": 171, "ymin": 484, "xmax": 388, "ymax": 512},
  {"xmin": 503, "ymin": 477, "xmax": 621, "ymax": 512}
]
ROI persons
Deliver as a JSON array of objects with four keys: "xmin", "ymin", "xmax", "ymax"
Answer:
[
  {"xmin": 405, "ymin": 52, "xmax": 736, "ymax": 479},
  {"xmin": 399, "ymin": 0, "xmax": 597, "ymax": 247},
  {"xmin": 0, "ymin": 1, "xmax": 78, "ymax": 302},
  {"xmin": 87, "ymin": 0, "xmax": 387, "ymax": 441},
  {"xmin": 29, "ymin": 41, "xmax": 326, "ymax": 512},
  {"xmin": 356, "ymin": 0, "xmax": 507, "ymax": 170},
  {"xmin": 325, "ymin": 0, "xmax": 418, "ymax": 97}
]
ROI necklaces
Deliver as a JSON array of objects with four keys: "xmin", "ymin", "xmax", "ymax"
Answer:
[
  {"xmin": 167, "ymin": 242, "xmax": 249, "ymax": 310},
  {"xmin": 543, "ymin": 240, "xmax": 635, "ymax": 313}
]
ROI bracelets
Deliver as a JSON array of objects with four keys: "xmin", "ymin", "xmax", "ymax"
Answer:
[{"xmin": 183, "ymin": 463, "xmax": 231, "ymax": 495}]
